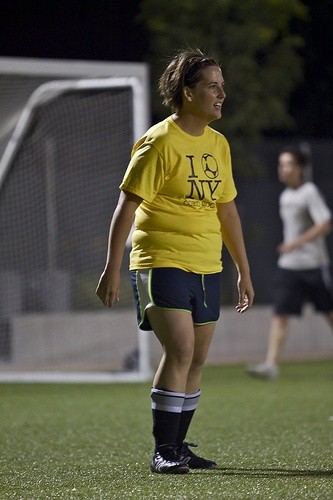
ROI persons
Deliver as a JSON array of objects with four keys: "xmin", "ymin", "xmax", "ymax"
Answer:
[
  {"xmin": 246, "ymin": 147, "xmax": 332, "ymax": 380},
  {"xmin": 95, "ymin": 45, "xmax": 254, "ymax": 474}
]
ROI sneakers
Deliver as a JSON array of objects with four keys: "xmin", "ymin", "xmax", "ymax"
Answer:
[
  {"xmin": 150, "ymin": 442, "xmax": 190, "ymax": 474},
  {"xmin": 178, "ymin": 442, "xmax": 217, "ymax": 470}
]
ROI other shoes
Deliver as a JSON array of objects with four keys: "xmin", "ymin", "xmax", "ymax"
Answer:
[{"xmin": 246, "ymin": 364, "xmax": 275, "ymax": 380}]
